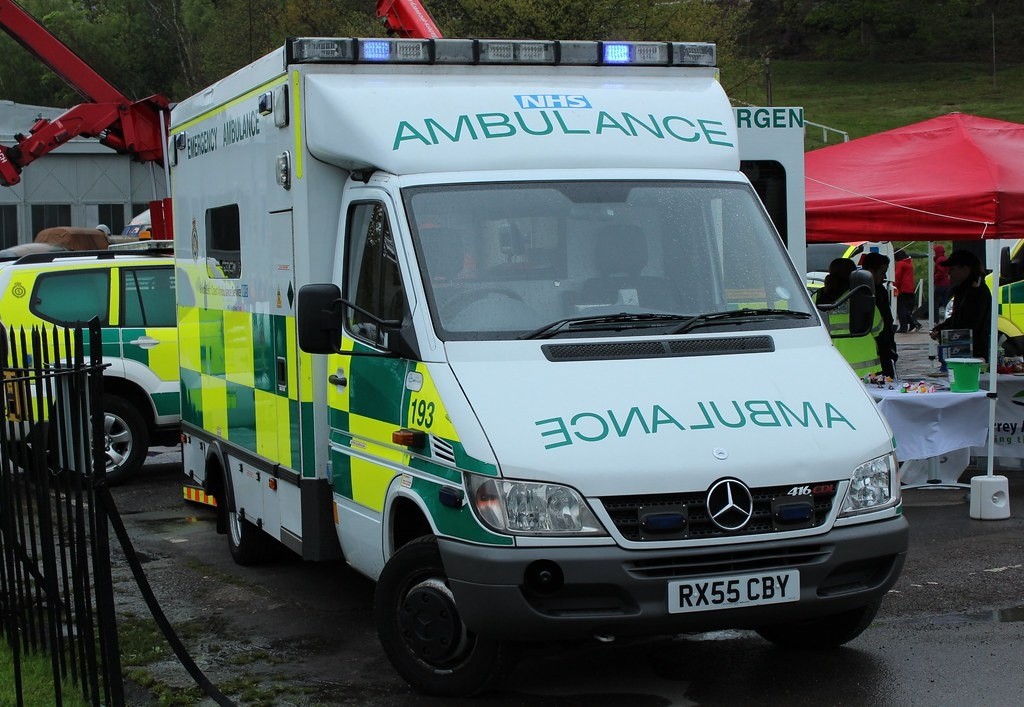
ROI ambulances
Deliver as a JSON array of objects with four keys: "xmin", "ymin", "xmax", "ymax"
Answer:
[
  {"xmin": 1, "ymin": 238, "xmax": 180, "ymax": 485},
  {"xmin": 164, "ymin": 35, "xmax": 911, "ymax": 697},
  {"xmin": 946, "ymin": 238, "xmax": 1024, "ymax": 358}
]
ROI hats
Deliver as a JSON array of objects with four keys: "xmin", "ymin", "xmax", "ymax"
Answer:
[
  {"xmin": 894, "ymin": 248, "xmax": 909, "ymax": 261},
  {"xmin": 939, "ymin": 250, "xmax": 982, "ymax": 267},
  {"xmin": 934, "ymin": 245, "xmax": 944, "ymax": 256},
  {"xmin": 96, "ymin": 223, "xmax": 110, "ymax": 235}
]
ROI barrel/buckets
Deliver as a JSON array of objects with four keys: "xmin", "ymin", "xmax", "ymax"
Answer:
[{"xmin": 945, "ymin": 358, "xmax": 983, "ymax": 393}]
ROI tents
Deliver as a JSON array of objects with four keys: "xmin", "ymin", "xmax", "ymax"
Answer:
[{"xmin": 805, "ymin": 111, "xmax": 1024, "ymax": 478}]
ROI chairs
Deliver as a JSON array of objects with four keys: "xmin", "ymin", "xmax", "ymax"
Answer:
[
  {"xmin": 389, "ymin": 228, "xmax": 469, "ymax": 324},
  {"xmin": 582, "ymin": 223, "xmax": 668, "ymax": 313}
]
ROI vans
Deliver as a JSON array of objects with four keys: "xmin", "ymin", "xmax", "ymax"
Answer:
[
  {"xmin": 807, "ymin": 234, "xmax": 903, "ymax": 330},
  {"xmin": 123, "ymin": 208, "xmax": 153, "ymax": 241}
]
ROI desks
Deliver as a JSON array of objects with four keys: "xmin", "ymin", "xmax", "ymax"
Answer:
[
  {"xmin": 900, "ymin": 373, "xmax": 1024, "ymax": 469},
  {"xmin": 865, "ymin": 382, "xmax": 990, "ymax": 492}
]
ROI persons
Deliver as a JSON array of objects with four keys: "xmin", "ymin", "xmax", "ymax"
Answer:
[
  {"xmin": 810, "ymin": 258, "xmax": 884, "ymax": 380},
  {"xmin": 859, "ymin": 253, "xmax": 899, "ymax": 379},
  {"xmin": 929, "ymin": 243, "xmax": 950, "ymax": 323},
  {"xmin": 929, "ymin": 249, "xmax": 993, "ymax": 372},
  {"xmin": 892, "ymin": 247, "xmax": 918, "ymax": 334}
]
